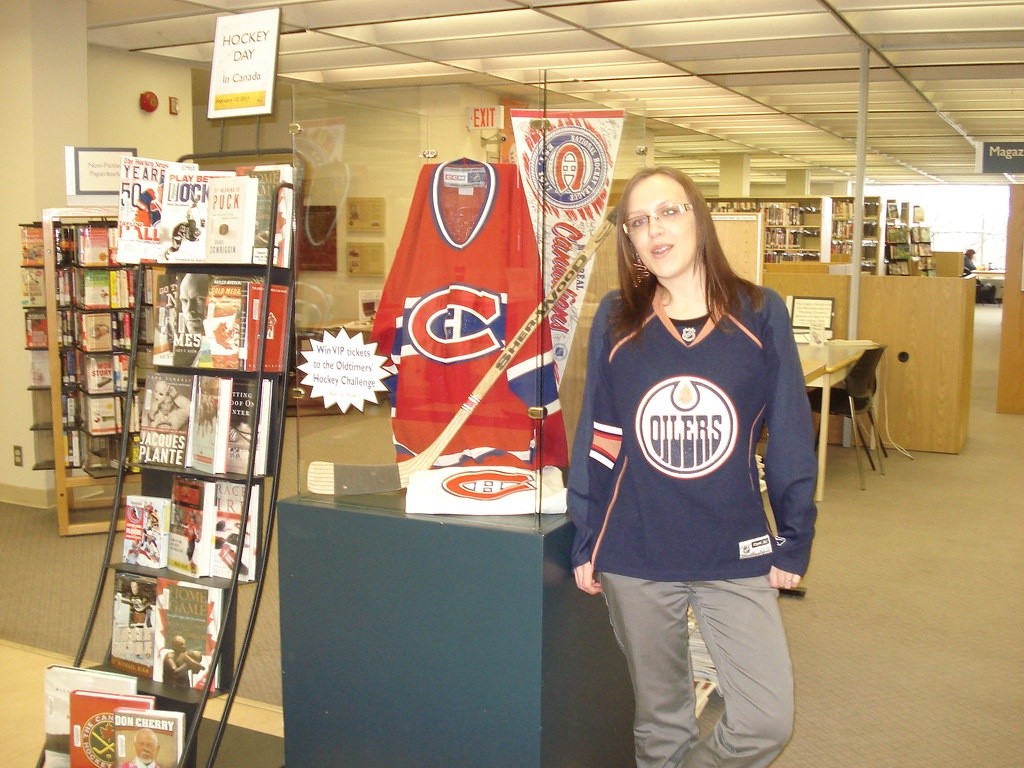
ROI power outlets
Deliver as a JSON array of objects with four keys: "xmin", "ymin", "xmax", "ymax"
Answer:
[{"xmin": 13, "ymin": 446, "xmax": 23, "ymax": 466}]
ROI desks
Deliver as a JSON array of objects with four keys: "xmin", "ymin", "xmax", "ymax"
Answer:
[{"xmin": 795, "ymin": 340, "xmax": 879, "ymax": 502}]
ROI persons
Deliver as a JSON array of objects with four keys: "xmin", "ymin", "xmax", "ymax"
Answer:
[
  {"xmin": 141, "ymin": 381, "xmax": 190, "ymax": 430},
  {"xmin": 177, "ymin": 273, "xmax": 212, "ymax": 335},
  {"xmin": 123, "ymin": 581, "xmax": 153, "ymax": 629},
  {"xmin": 964, "ymin": 249, "xmax": 976, "ymax": 276},
  {"xmin": 161, "ymin": 636, "xmax": 192, "ymax": 687},
  {"xmin": 566, "ymin": 168, "xmax": 817, "ymax": 767}
]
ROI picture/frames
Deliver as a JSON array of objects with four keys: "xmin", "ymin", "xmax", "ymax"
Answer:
[{"xmin": 791, "ymin": 294, "xmax": 834, "ymax": 330}]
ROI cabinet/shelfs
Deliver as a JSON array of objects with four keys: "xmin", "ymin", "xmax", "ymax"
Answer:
[
  {"xmin": 18, "ymin": 146, "xmax": 314, "ymax": 768},
  {"xmin": 700, "ymin": 195, "xmax": 938, "ymax": 276}
]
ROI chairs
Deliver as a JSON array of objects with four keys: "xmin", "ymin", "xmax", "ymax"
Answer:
[{"xmin": 807, "ymin": 345, "xmax": 889, "ymax": 490}]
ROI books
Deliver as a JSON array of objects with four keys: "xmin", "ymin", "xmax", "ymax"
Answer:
[
  {"xmin": 20, "ymin": 223, "xmax": 164, "ymax": 474},
  {"xmin": 155, "ymin": 275, "xmax": 291, "ymax": 371},
  {"xmin": 122, "ymin": 476, "xmax": 262, "ymax": 584},
  {"xmin": 707, "ymin": 202, "xmax": 936, "ymax": 278},
  {"xmin": 116, "ymin": 156, "xmax": 293, "ymax": 270},
  {"xmin": 140, "ymin": 370, "xmax": 274, "ymax": 477},
  {"xmin": 107, "ymin": 573, "xmax": 229, "ymax": 693},
  {"xmin": 42, "ymin": 664, "xmax": 187, "ymax": 768}
]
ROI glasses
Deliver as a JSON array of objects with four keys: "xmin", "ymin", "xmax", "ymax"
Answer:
[{"xmin": 622, "ymin": 204, "xmax": 694, "ymax": 234}]
